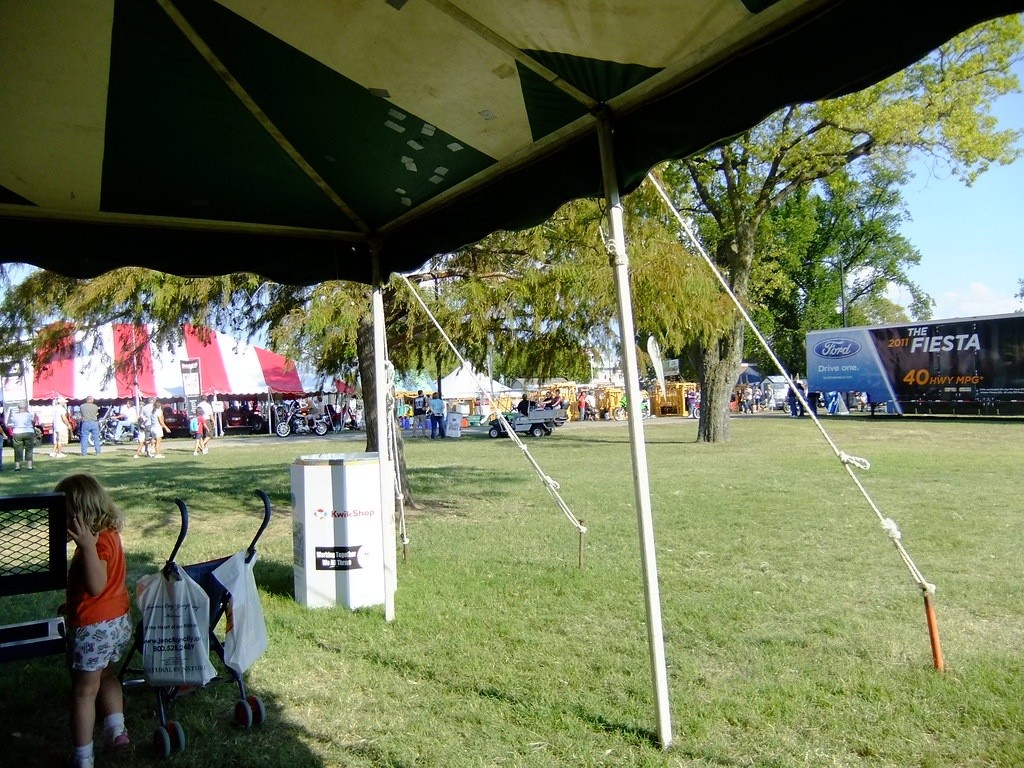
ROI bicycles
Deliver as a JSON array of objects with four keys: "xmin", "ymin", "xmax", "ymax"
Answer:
[{"xmin": 614, "ymin": 405, "xmax": 648, "ymax": 421}]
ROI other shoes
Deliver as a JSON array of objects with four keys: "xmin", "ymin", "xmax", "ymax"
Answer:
[
  {"xmin": 411, "ymin": 436, "xmax": 415, "ymax": 438},
  {"xmin": 133, "ymin": 455, "xmax": 140, "ymax": 458},
  {"xmin": 50, "ymin": 452, "xmax": 56, "ymax": 457},
  {"xmin": 154, "ymin": 454, "xmax": 165, "ymax": 459},
  {"xmin": 311, "ymin": 425, "xmax": 317, "ymax": 429},
  {"xmin": 71, "ymin": 755, "xmax": 94, "ymax": 768},
  {"xmin": 15, "ymin": 467, "xmax": 20, "ymax": 471},
  {"xmin": 194, "ymin": 452, "xmax": 199, "ymax": 456},
  {"xmin": 422, "ymin": 436, "xmax": 426, "ymax": 439},
  {"xmin": 28, "ymin": 467, "xmax": 32, "ymax": 470},
  {"xmin": 202, "ymin": 449, "xmax": 209, "ymax": 454},
  {"xmin": 302, "ymin": 425, "xmax": 309, "ymax": 430},
  {"xmin": 792, "ymin": 415, "xmax": 798, "ymax": 418},
  {"xmin": 55, "ymin": 453, "xmax": 67, "ymax": 458},
  {"xmin": 103, "ymin": 724, "xmax": 130, "ymax": 748},
  {"xmin": 111, "ymin": 437, "xmax": 120, "ymax": 441}
]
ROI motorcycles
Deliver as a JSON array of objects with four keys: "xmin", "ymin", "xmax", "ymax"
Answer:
[
  {"xmin": 88, "ymin": 417, "xmax": 138, "ymax": 446},
  {"xmin": 276, "ymin": 408, "xmax": 328, "ymax": 436}
]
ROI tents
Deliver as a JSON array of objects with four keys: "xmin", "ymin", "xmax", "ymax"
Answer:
[
  {"xmin": 417, "ymin": 361, "xmax": 524, "ymax": 414},
  {"xmin": 0, "ymin": 0, "xmax": 1024, "ymax": 748},
  {"xmin": 0, "ymin": 321, "xmax": 356, "ymax": 433}
]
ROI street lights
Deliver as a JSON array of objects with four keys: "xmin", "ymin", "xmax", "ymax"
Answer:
[{"xmin": 802, "ymin": 260, "xmax": 846, "ymax": 327}]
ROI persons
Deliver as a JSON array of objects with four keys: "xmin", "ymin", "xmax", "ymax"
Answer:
[
  {"xmin": 0, "ymin": 401, "xmax": 7, "ymax": 471},
  {"xmin": 134, "ymin": 396, "xmax": 171, "ymax": 458},
  {"xmin": 80, "ymin": 396, "xmax": 101, "ymax": 456},
  {"xmin": 788, "ymin": 381, "xmax": 817, "ymax": 416},
  {"xmin": 552, "ymin": 390, "xmax": 561, "ymax": 410},
  {"xmin": 12, "ymin": 401, "xmax": 34, "ymax": 471},
  {"xmin": 50, "ymin": 398, "xmax": 72, "ymax": 458},
  {"xmin": 52, "ymin": 474, "xmax": 130, "ymax": 768},
  {"xmin": 412, "ymin": 390, "xmax": 429, "ymax": 438},
  {"xmin": 111, "ymin": 400, "xmax": 137, "ymax": 441},
  {"xmin": 512, "ymin": 393, "xmax": 535, "ymax": 430},
  {"xmin": 540, "ymin": 392, "xmax": 553, "ymax": 410},
  {"xmin": 312, "ymin": 397, "xmax": 324, "ymax": 429},
  {"xmin": 736, "ymin": 382, "xmax": 762, "ymax": 414},
  {"xmin": 578, "ymin": 392, "xmax": 586, "ymax": 422},
  {"xmin": 197, "ymin": 394, "xmax": 214, "ymax": 452},
  {"xmin": 302, "ymin": 400, "xmax": 316, "ymax": 429},
  {"xmin": 429, "ymin": 392, "xmax": 447, "ymax": 439},
  {"xmin": 193, "ymin": 407, "xmax": 209, "ymax": 455},
  {"xmin": 687, "ymin": 386, "xmax": 697, "ymax": 418},
  {"xmin": 828, "ymin": 391, "xmax": 865, "ymax": 415},
  {"xmin": 290, "ymin": 398, "xmax": 300, "ymax": 414},
  {"xmin": 766, "ymin": 384, "xmax": 776, "ymax": 411},
  {"xmin": 585, "ymin": 390, "xmax": 595, "ymax": 421}
]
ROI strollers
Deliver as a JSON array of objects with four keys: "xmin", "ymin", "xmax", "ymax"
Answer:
[{"xmin": 118, "ymin": 488, "xmax": 271, "ymax": 757}]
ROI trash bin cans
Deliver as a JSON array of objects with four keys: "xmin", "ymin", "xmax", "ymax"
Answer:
[
  {"xmin": 289, "ymin": 453, "xmax": 395, "ymax": 610},
  {"xmin": 445, "ymin": 411, "xmax": 463, "ymax": 437}
]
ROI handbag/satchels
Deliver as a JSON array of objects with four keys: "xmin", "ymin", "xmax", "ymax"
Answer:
[
  {"xmin": 212, "ymin": 548, "xmax": 266, "ymax": 674},
  {"xmin": 135, "ymin": 563, "xmax": 218, "ymax": 687}
]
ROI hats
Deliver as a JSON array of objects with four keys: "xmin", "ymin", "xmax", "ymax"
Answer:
[{"xmin": 58, "ymin": 397, "xmax": 68, "ymax": 402}]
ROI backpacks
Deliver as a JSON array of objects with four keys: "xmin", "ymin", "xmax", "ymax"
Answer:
[{"xmin": 190, "ymin": 415, "xmax": 201, "ymax": 432}]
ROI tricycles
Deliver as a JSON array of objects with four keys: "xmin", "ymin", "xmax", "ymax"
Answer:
[{"xmin": 489, "ymin": 407, "xmax": 568, "ymax": 438}]
